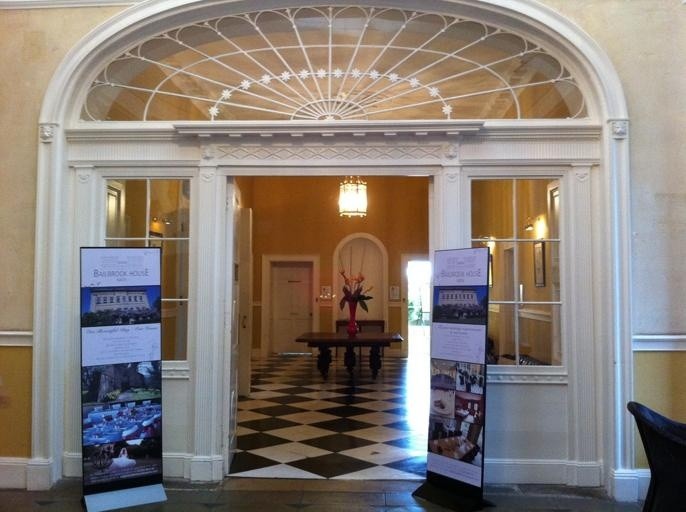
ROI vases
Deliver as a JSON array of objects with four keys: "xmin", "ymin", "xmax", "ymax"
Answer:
[{"xmin": 344, "ymin": 298, "xmax": 359, "ymax": 339}]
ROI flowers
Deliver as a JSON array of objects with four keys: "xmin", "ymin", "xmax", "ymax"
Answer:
[{"xmin": 335, "ymin": 242, "xmax": 377, "ymax": 314}]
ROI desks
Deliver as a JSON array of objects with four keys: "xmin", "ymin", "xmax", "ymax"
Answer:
[{"xmin": 294, "ymin": 329, "xmax": 405, "ymax": 381}]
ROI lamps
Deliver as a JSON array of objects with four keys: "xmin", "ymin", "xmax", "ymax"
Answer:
[
  {"xmin": 525, "ymin": 215, "xmax": 542, "ymax": 231},
  {"xmin": 338, "ymin": 175, "xmax": 367, "ymax": 218}
]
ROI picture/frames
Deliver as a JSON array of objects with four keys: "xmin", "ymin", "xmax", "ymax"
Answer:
[
  {"xmin": 532, "ymin": 238, "xmax": 547, "ymax": 288},
  {"xmin": 487, "ymin": 253, "xmax": 494, "ymax": 289},
  {"xmin": 149, "ymin": 231, "xmax": 164, "ymax": 251}
]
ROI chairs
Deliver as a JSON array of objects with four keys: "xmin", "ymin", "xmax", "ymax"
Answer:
[{"xmin": 624, "ymin": 399, "xmax": 686, "ymax": 512}]
